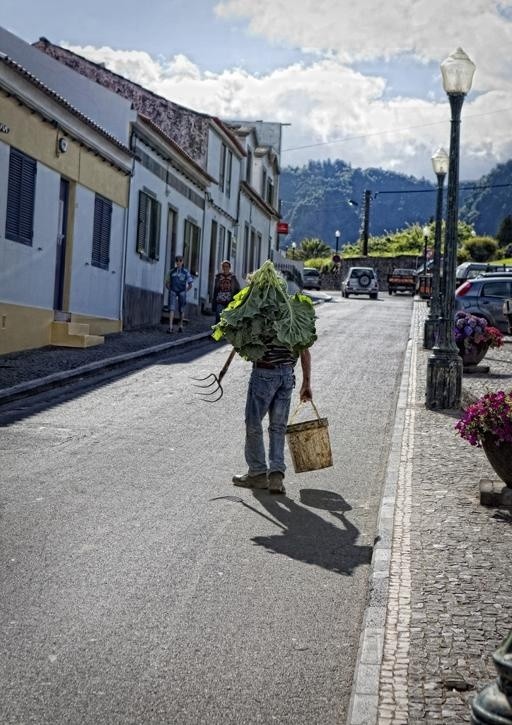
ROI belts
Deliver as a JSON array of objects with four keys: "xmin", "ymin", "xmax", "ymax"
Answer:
[{"xmin": 252, "ymin": 362, "xmax": 296, "ymax": 369}]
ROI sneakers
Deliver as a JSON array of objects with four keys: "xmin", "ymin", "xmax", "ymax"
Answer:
[
  {"xmin": 232, "ymin": 473, "xmax": 270, "ymax": 489},
  {"xmin": 166, "ymin": 328, "xmax": 174, "ymax": 334},
  {"xmin": 268, "ymin": 472, "xmax": 286, "ymax": 494},
  {"xmin": 178, "ymin": 325, "xmax": 183, "ymax": 332}
]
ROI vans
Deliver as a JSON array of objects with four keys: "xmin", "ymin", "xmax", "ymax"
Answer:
[{"xmin": 456, "ymin": 262, "xmax": 486, "ymax": 279}]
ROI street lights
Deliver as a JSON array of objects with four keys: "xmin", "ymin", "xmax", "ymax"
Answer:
[
  {"xmin": 423, "ymin": 226, "xmax": 431, "ymax": 275},
  {"xmin": 424, "ymin": 44, "xmax": 477, "ymax": 411},
  {"xmin": 423, "ymin": 146, "xmax": 450, "ymax": 349},
  {"xmin": 335, "ymin": 230, "xmax": 341, "ymax": 252},
  {"xmin": 291, "ymin": 242, "xmax": 296, "ymax": 260}
]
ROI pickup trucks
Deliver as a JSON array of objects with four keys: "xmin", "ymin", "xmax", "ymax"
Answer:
[
  {"xmin": 385, "ymin": 267, "xmax": 420, "ymax": 296},
  {"xmin": 419, "ymin": 261, "xmax": 512, "ymax": 303}
]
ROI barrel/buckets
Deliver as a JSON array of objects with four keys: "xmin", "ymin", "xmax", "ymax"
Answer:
[{"xmin": 282, "ymin": 400, "xmax": 334, "ymax": 474}]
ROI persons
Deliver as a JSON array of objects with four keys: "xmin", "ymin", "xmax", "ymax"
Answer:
[
  {"xmin": 233, "ymin": 281, "xmax": 312, "ymax": 494},
  {"xmin": 165, "ymin": 256, "xmax": 193, "ymax": 334},
  {"xmin": 211, "ymin": 260, "xmax": 241, "ymax": 333}
]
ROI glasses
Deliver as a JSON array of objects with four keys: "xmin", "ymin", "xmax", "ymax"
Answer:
[{"xmin": 175, "ymin": 259, "xmax": 182, "ymax": 263}]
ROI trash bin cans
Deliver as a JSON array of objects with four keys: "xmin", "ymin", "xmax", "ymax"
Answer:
[{"xmin": 420, "ymin": 276, "xmax": 432, "ymax": 299}]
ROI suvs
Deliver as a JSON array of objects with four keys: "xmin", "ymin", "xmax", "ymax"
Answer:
[{"xmin": 341, "ymin": 265, "xmax": 379, "ymax": 300}]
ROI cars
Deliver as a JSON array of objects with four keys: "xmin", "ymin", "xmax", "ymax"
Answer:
[
  {"xmin": 456, "ymin": 276, "xmax": 512, "ymax": 337},
  {"xmin": 301, "ymin": 267, "xmax": 323, "ymax": 291}
]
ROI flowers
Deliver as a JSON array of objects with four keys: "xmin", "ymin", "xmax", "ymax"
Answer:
[
  {"xmin": 455, "ymin": 391, "xmax": 512, "ymax": 448},
  {"xmin": 451, "ymin": 311, "xmax": 504, "ymax": 350}
]
